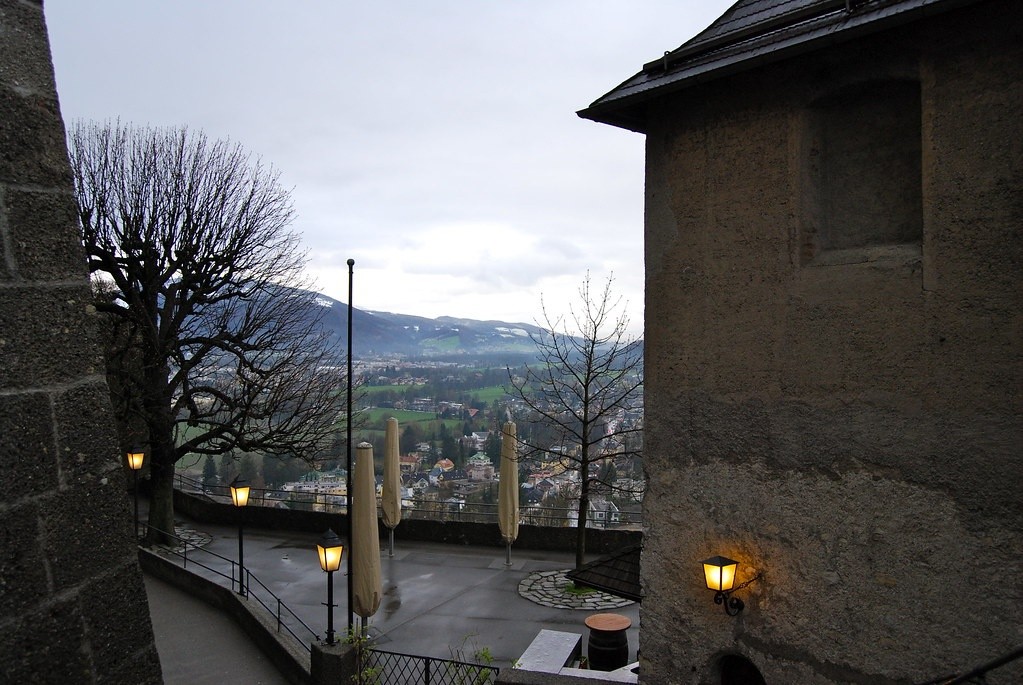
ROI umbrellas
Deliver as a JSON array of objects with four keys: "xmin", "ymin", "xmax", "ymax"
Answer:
[
  {"xmin": 564, "ymin": 538, "xmax": 641, "ymax": 606},
  {"xmin": 349, "ymin": 440, "xmax": 382, "ymax": 639},
  {"xmin": 381, "ymin": 417, "xmax": 403, "ymax": 562},
  {"xmin": 497, "ymin": 420, "xmax": 521, "ymax": 568}
]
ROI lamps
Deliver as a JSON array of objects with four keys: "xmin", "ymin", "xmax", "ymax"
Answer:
[{"xmin": 697, "ymin": 556, "xmax": 764, "ymax": 617}]
